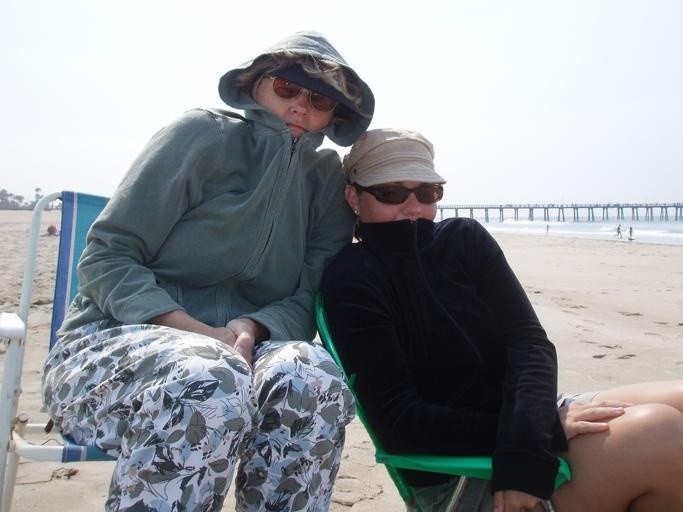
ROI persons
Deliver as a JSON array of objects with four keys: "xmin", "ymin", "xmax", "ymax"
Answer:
[
  {"xmin": 320, "ymin": 128, "xmax": 681, "ymax": 511},
  {"xmin": 42, "ymin": 31, "xmax": 372, "ymax": 510},
  {"xmin": 616, "ymin": 224, "xmax": 624, "ymax": 239}
]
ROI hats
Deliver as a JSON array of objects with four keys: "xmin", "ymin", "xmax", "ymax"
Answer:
[
  {"xmin": 348, "ymin": 129, "xmax": 446, "ymax": 187},
  {"xmin": 272, "ymin": 60, "xmax": 372, "ymax": 121}
]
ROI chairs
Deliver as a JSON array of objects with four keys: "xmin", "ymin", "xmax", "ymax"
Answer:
[
  {"xmin": 1, "ymin": 191, "xmax": 118, "ymax": 510},
  {"xmin": 314, "ymin": 291, "xmax": 571, "ymax": 512}
]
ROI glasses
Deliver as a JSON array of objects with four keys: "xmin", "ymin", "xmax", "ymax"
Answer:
[
  {"xmin": 273, "ymin": 75, "xmax": 337, "ymax": 112},
  {"xmin": 353, "ymin": 182, "xmax": 443, "ymax": 203}
]
